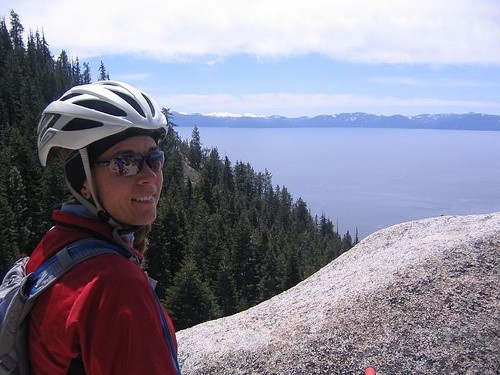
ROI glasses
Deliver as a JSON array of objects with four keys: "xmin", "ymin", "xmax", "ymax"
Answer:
[{"xmin": 93, "ymin": 150, "xmax": 165, "ymax": 176}]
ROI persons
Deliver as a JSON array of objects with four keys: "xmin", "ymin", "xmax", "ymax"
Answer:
[{"xmin": 25, "ymin": 80, "xmax": 181, "ymax": 375}]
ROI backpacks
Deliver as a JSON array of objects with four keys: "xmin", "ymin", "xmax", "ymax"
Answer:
[{"xmin": 0, "ymin": 236, "xmax": 181, "ymax": 375}]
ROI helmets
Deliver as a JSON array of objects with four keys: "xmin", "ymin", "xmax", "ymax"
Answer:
[{"xmin": 36, "ymin": 80, "xmax": 167, "ymax": 166}]
